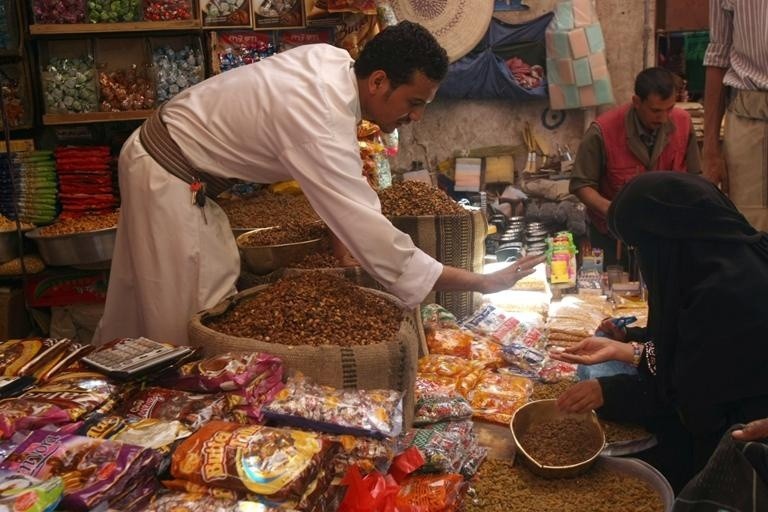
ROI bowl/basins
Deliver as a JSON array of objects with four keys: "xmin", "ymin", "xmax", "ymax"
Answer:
[
  {"xmin": 510, "ymin": 398, "xmax": 674, "ymax": 512},
  {"xmin": 0, "ymin": 217, "xmax": 117, "ymax": 267},
  {"xmin": 237, "ymin": 226, "xmax": 321, "ymax": 275}
]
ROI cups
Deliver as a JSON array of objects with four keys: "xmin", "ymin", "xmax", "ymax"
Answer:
[
  {"xmin": 492, "ymin": 214, "xmax": 549, "ymax": 252},
  {"xmin": 607, "ymin": 263, "xmax": 624, "ymax": 290}
]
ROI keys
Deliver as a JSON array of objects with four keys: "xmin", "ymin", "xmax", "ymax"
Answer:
[{"xmin": 191, "ymin": 173, "xmax": 210, "ymax": 222}]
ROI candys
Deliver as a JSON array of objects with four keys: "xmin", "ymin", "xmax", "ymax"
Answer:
[{"xmin": 0, "ymin": 0, "xmax": 305, "ymax": 134}]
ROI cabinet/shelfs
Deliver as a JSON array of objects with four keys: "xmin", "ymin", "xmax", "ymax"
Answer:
[{"xmin": 0, "ymin": 1, "xmax": 343, "ymax": 132}]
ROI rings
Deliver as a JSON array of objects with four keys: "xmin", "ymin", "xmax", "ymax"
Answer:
[{"xmin": 515, "ymin": 266, "xmax": 523, "ymax": 274}]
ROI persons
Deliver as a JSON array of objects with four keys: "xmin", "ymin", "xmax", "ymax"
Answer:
[
  {"xmin": 570, "ymin": 65, "xmax": 703, "ymax": 272},
  {"xmin": 91, "ymin": 19, "xmax": 548, "ymax": 349},
  {"xmin": 701, "ymin": 1, "xmax": 767, "ymax": 235},
  {"xmin": 549, "ymin": 170, "xmax": 767, "ymax": 501}
]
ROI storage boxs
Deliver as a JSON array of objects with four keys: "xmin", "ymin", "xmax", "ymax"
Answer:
[{"xmin": 0, "ymin": 270, "xmax": 108, "ymax": 344}]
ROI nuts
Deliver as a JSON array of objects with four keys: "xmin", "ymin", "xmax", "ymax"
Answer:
[
  {"xmin": 456, "ymin": 376, "xmax": 663, "ymax": 512},
  {"xmin": 0, "ymin": 179, "xmax": 470, "ymax": 348}
]
